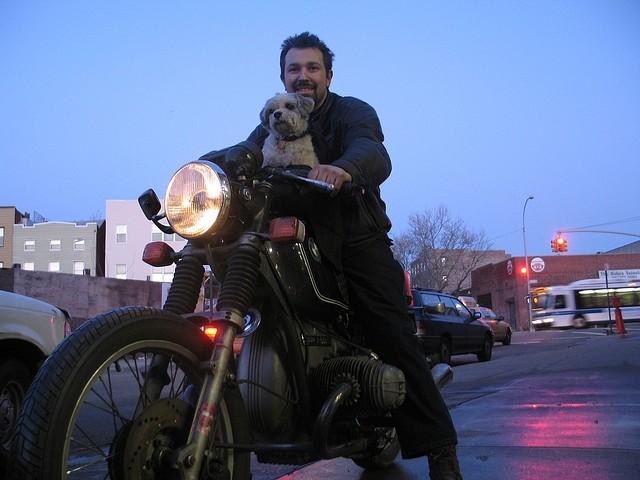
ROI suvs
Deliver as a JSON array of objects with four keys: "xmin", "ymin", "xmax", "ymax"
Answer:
[
  {"xmin": 406, "ymin": 288, "xmax": 495, "ymax": 368},
  {"xmin": 1, "ymin": 291, "xmax": 75, "ymax": 480}
]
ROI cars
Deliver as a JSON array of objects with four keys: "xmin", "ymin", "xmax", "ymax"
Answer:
[{"xmin": 467, "ymin": 305, "xmax": 513, "ymax": 346}]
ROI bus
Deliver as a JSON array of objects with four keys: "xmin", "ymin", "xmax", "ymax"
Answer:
[{"xmin": 525, "ymin": 279, "xmax": 640, "ymax": 330}]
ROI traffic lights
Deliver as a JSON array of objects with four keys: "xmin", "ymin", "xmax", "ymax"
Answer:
[
  {"xmin": 559, "ymin": 239, "xmax": 569, "ymax": 253},
  {"xmin": 551, "ymin": 239, "xmax": 560, "ymax": 252},
  {"xmin": 557, "ymin": 238, "xmax": 564, "ymax": 245}
]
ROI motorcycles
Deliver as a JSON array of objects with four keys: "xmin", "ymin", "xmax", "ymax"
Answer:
[{"xmin": 12, "ymin": 142, "xmax": 453, "ymax": 479}]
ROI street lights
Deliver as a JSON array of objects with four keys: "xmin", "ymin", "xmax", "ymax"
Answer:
[{"xmin": 523, "ymin": 196, "xmax": 534, "ymax": 332}]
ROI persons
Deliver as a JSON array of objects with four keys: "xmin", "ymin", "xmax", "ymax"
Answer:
[{"xmin": 242, "ymin": 34, "xmax": 463, "ymax": 479}]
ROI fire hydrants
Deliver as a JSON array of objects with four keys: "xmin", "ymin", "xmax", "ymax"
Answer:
[{"xmin": 612, "ymin": 291, "xmax": 627, "ymax": 334}]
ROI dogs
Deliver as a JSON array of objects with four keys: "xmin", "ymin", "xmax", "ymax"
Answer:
[{"xmin": 260, "ymin": 92, "xmax": 321, "ymax": 169}]
